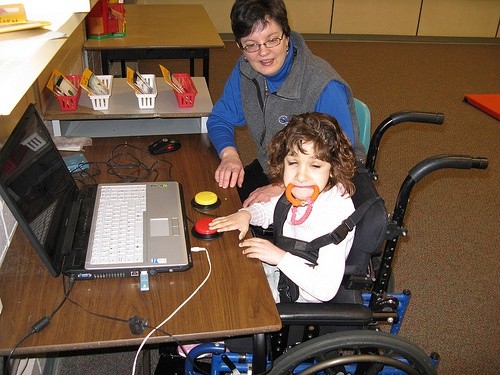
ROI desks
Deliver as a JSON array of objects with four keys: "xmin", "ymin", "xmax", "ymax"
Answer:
[
  {"xmin": 0, "ymin": 137, "xmax": 282, "ymax": 375},
  {"xmin": 83, "ymin": 4, "xmax": 225, "ymax": 89},
  {"xmin": 43, "ymin": 77, "xmax": 213, "ymax": 137}
]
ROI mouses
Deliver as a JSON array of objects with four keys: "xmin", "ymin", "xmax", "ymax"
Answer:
[{"xmin": 149, "ymin": 138, "xmax": 182, "ymax": 154}]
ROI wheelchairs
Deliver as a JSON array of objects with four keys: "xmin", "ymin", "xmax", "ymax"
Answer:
[{"xmin": 188, "ymin": 111, "xmax": 489, "ymax": 373}]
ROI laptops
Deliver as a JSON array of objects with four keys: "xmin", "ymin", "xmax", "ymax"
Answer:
[{"xmin": 1, "ymin": 102, "xmax": 194, "ymax": 279}]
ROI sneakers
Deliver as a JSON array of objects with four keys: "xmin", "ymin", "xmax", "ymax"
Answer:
[{"xmin": 178, "ymin": 340, "xmax": 224, "ymax": 359}]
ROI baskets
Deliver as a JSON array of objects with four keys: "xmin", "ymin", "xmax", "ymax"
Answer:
[
  {"xmin": 172, "ymin": 72, "xmax": 197, "ymax": 108},
  {"xmin": 134, "ymin": 73, "xmax": 159, "ymax": 109},
  {"xmin": 88, "ymin": 74, "xmax": 113, "ymax": 110},
  {"xmin": 57, "ymin": 74, "xmax": 83, "ymax": 111}
]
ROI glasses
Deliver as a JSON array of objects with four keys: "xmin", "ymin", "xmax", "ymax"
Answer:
[{"xmin": 239, "ymin": 28, "xmax": 286, "ymax": 53}]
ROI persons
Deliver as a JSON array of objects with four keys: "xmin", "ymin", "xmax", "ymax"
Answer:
[
  {"xmin": 205, "ymin": 0, "xmax": 363, "ymax": 207},
  {"xmin": 153, "ymin": 112, "xmax": 357, "ymax": 375}
]
